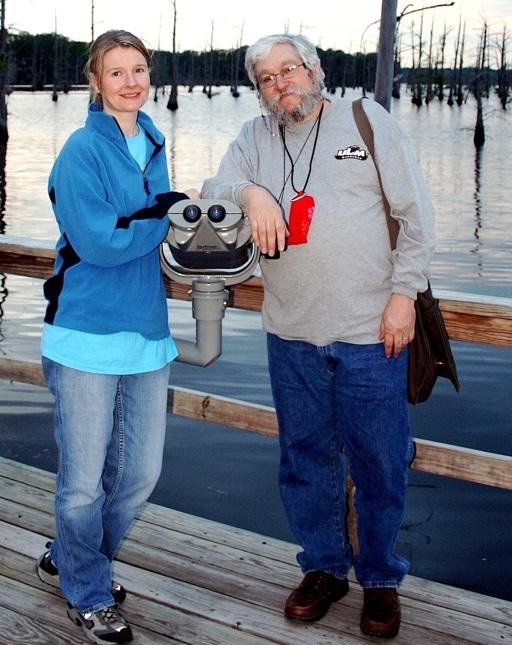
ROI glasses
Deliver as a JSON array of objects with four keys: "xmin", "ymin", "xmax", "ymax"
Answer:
[{"xmin": 258, "ymin": 63, "xmax": 304, "ymax": 90}]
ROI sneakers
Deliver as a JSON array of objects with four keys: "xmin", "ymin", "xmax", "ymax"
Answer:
[
  {"xmin": 65, "ymin": 600, "xmax": 134, "ymax": 644},
  {"xmin": 35, "ymin": 540, "xmax": 126, "ymax": 604}
]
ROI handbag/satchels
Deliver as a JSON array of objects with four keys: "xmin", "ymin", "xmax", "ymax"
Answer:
[{"xmin": 407, "ymin": 280, "xmax": 461, "ymax": 403}]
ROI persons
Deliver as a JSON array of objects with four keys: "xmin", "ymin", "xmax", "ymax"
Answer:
[
  {"xmin": 36, "ymin": 31, "xmax": 202, "ymax": 645},
  {"xmin": 200, "ymin": 35, "xmax": 435, "ymax": 638}
]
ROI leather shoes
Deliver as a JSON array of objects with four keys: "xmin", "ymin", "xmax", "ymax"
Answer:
[
  {"xmin": 359, "ymin": 584, "xmax": 402, "ymax": 640},
  {"xmin": 284, "ymin": 571, "xmax": 349, "ymax": 623}
]
